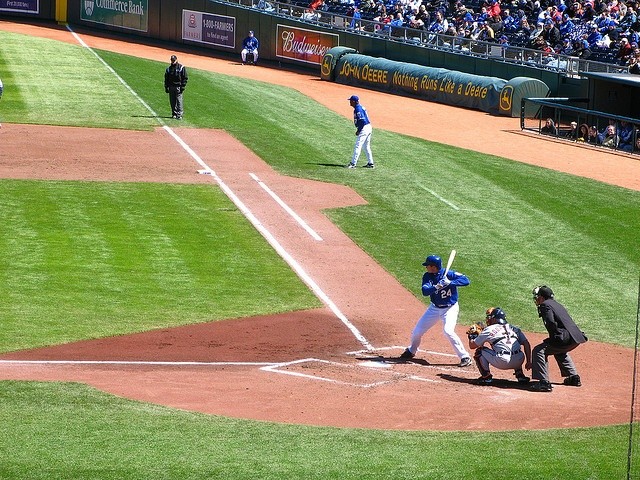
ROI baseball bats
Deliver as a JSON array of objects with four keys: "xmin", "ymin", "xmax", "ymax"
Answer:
[{"xmin": 439, "ymin": 249, "xmax": 456, "ymax": 288}]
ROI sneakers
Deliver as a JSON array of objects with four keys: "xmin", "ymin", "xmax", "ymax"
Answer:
[
  {"xmin": 518, "ymin": 375, "xmax": 530, "ymax": 386},
  {"xmin": 471, "ymin": 374, "xmax": 492, "ymax": 386},
  {"xmin": 564, "ymin": 375, "xmax": 581, "ymax": 386},
  {"xmin": 527, "ymin": 379, "xmax": 553, "ymax": 391},
  {"xmin": 458, "ymin": 358, "xmax": 472, "ymax": 367},
  {"xmin": 242, "ymin": 62, "xmax": 246, "ymax": 65},
  {"xmin": 251, "ymin": 62, "xmax": 256, "ymax": 66},
  {"xmin": 363, "ymin": 163, "xmax": 373, "ymax": 168},
  {"xmin": 396, "ymin": 348, "xmax": 415, "ymax": 363},
  {"xmin": 344, "ymin": 163, "xmax": 356, "ymax": 170}
]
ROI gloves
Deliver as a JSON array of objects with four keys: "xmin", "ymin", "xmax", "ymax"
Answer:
[{"xmin": 434, "ymin": 275, "xmax": 451, "ymax": 290}]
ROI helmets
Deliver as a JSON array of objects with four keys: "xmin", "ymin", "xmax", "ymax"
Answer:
[
  {"xmin": 532, "ymin": 285, "xmax": 554, "ymax": 307},
  {"xmin": 485, "ymin": 307, "xmax": 507, "ymax": 326},
  {"xmin": 422, "ymin": 255, "xmax": 441, "ymax": 268}
]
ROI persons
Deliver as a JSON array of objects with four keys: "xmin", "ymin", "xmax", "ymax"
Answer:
[
  {"xmin": 241, "ymin": 30, "xmax": 258, "ymax": 65},
  {"xmin": 542, "ymin": 118, "xmax": 558, "ymax": 136},
  {"xmin": 399, "ymin": 256, "xmax": 473, "ymax": 367},
  {"xmin": 469, "ymin": 307, "xmax": 532, "ymax": 383},
  {"xmin": 345, "ymin": 95, "xmax": 375, "ymax": 168},
  {"xmin": 164, "ymin": 55, "xmax": 187, "ymax": 120},
  {"xmin": 289, "ymin": 0, "xmax": 640, "ymax": 74},
  {"xmin": 253, "ymin": 0, "xmax": 274, "ymax": 11},
  {"xmin": 566, "ymin": 119, "xmax": 640, "ymax": 155},
  {"xmin": 524, "ymin": 285, "xmax": 588, "ymax": 392}
]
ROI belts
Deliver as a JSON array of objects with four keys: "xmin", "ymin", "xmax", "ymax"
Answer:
[
  {"xmin": 435, "ymin": 304, "xmax": 454, "ymax": 309},
  {"xmin": 497, "ymin": 349, "xmax": 521, "ymax": 355}
]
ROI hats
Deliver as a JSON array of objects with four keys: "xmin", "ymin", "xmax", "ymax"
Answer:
[
  {"xmin": 171, "ymin": 55, "xmax": 176, "ymax": 61},
  {"xmin": 347, "ymin": 95, "xmax": 359, "ymax": 101},
  {"xmin": 249, "ymin": 31, "xmax": 254, "ymax": 34}
]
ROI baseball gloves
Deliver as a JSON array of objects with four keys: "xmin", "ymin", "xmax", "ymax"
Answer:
[{"xmin": 466, "ymin": 321, "xmax": 487, "ymax": 340}]
